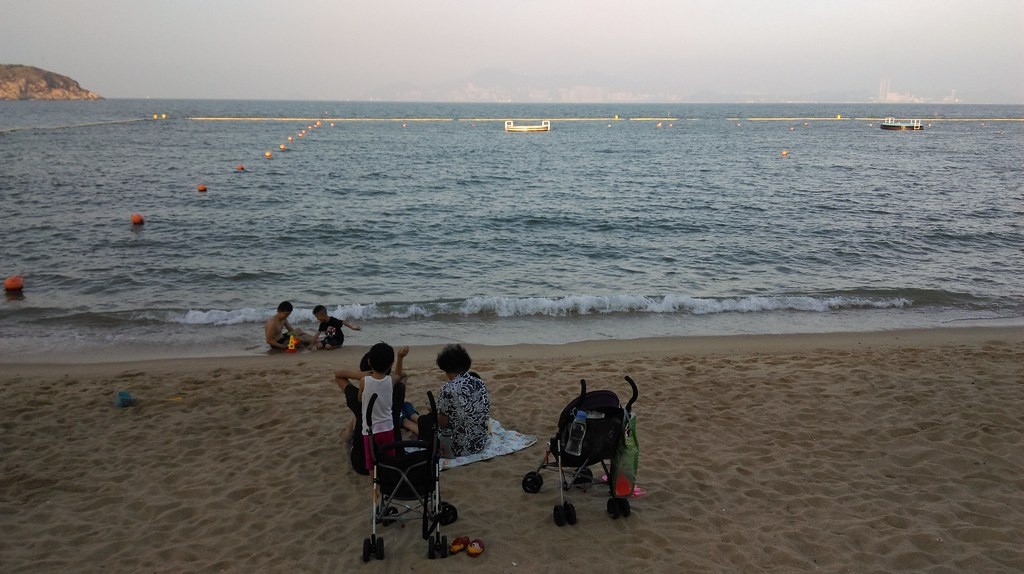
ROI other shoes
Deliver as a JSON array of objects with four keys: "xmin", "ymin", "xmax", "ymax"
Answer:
[
  {"xmin": 449, "ymin": 536, "xmax": 470, "ymax": 555},
  {"xmin": 467, "ymin": 539, "xmax": 485, "ymax": 558},
  {"xmin": 403, "ymin": 402, "xmax": 419, "ymax": 420},
  {"xmin": 399, "ymin": 411, "xmax": 407, "ymax": 426}
]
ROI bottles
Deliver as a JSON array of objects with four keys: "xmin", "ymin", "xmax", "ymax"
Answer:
[
  {"xmin": 439, "ymin": 428, "xmax": 452, "ymax": 458},
  {"xmin": 565, "ymin": 411, "xmax": 588, "ymax": 456},
  {"xmin": 617, "ymin": 437, "xmax": 633, "ymax": 495}
]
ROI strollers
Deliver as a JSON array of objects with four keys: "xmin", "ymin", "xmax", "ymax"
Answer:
[
  {"xmin": 361, "ymin": 392, "xmax": 457, "ymax": 561},
  {"xmin": 521, "ymin": 374, "xmax": 640, "ymax": 526}
]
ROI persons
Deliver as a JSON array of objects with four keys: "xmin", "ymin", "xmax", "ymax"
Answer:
[
  {"xmin": 399, "ymin": 344, "xmax": 491, "ymax": 460},
  {"xmin": 307, "ymin": 305, "xmax": 362, "ymax": 351},
  {"xmin": 264, "ymin": 301, "xmax": 325, "ymax": 352},
  {"xmin": 358, "ymin": 341, "xmax": 409, "ymax": 503},
  {"xmin": 334, "ymin": 351, "xmax": 407, "ymax": 475}
]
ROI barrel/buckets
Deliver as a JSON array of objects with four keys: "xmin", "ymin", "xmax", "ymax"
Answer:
[{"xmin": 116, "ymin": 391, "xmax": 132, "ymax": 408}]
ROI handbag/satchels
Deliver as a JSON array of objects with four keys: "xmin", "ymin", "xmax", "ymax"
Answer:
[{"xmin": 610, "ymin": 405, "xmax": 639, "ymax": 498}]
